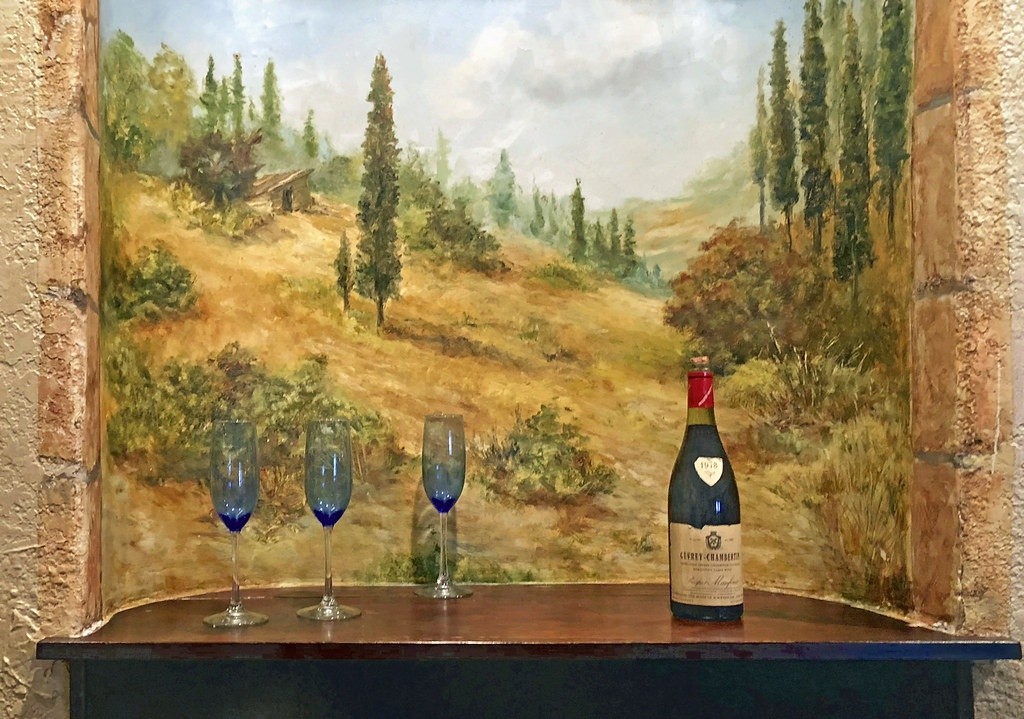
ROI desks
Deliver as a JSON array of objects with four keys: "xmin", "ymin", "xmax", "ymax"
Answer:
[{"xmin": 35, "ymin": 578, "xmax": 1023, "ymax": 719}]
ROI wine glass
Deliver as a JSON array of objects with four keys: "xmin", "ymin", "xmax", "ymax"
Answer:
[
  {"xmin": 413, "ymin": 414, "xmax": 473, "ymax": 599},
  {"xmin": 203, "ymin": 421, "xmax": 269, "ymax": 627},
  {"xmin": 297, "ymin": 419, "xmax": 362, "ymax": 621}
]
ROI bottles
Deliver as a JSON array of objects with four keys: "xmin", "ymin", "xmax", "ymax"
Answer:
[{"xmin": 668, "ymin": 356, "xmax": 744, "ymax": 621}]
